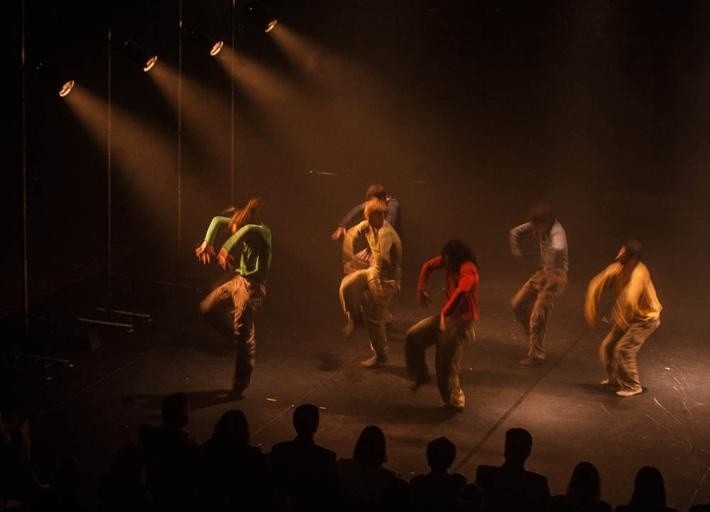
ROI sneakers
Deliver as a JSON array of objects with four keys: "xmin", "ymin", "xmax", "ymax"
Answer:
[
  {"xmin": 229, "ymin": 387, "xmax": 246, "ymax": 400},
  {"xmin": 359, "ymin": 355, "xmax": 391, "ymax": 368},
  {"xmin": 438, "ymin": 404, "xmax": 463, "ymax": 413}
]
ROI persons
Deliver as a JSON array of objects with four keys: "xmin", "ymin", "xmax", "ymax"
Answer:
[
  {"xmin": 403, "ymin": 238, "xmax": 482, "ymax": 413},
  {"xmin": 330, "ymin": 184, "xmax": 401, "ymax": 323},
  {"xmin": 1, "ymin": 391, "xmax": 416, "ymax": 511},
  {"xmin": 584, "ymin": 238, "xmax": 663, "ymax": 397},
  {"xmin": 195, "ymin": 196, "xmax": 272, "ymax": 400},
  {"xmin": 409, "ymin": 427, "xmax": 676, "ymax": 512},
  {"xmin": 509, "ymin": 203, "xmax": 568, "ymax": 363},
  {"xmin": 339, "ymin": 198, "xmax": 402, "ymax": 368}
]
